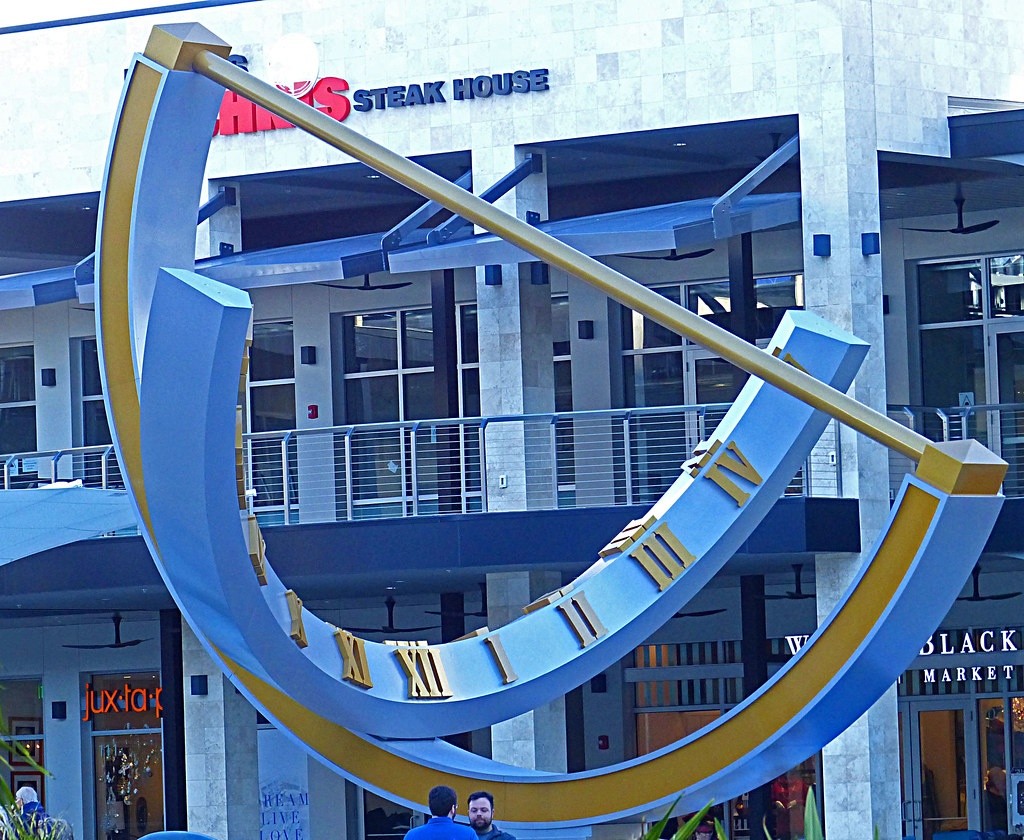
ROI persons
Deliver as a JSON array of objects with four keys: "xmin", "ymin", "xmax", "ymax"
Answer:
[
  {"xmin": 772, "ymin": 764, "xmax": 807, "ymax": 840},
  {"xmin": 403, "ymin": 786, "xmax": 517, "ymax": 840},
  {"xmin": 987, "ymin": 759, "xmax": 1008, "ymax": 831},
  {"xmin": 6, "ymin": 786, "xmax": 55, "ymax": 840}
]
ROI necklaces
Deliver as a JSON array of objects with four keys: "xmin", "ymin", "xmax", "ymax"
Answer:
[{"xmin": 782, "ymin": 784, "xmax": 795, "ymax": 801}]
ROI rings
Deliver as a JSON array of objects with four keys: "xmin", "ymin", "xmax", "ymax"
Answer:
[{"xmin": 790, "ymin": 806, "xmax": 791, "ymax": 808}]
